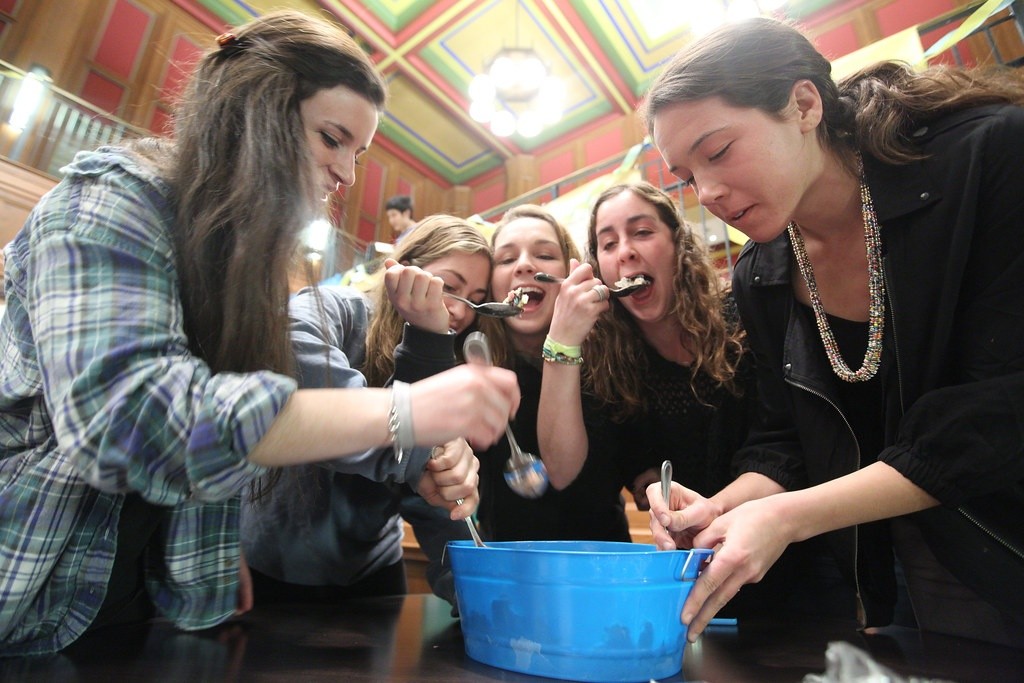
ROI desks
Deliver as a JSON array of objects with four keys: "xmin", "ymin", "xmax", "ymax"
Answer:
[{"xmin": 0, "ymin": 592, "xmax": 1024, "ymax": 683}]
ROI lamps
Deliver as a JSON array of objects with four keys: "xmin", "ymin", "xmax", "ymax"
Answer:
[{"xmin": 465, "ymin": 1, "xmax": 570, "ymax": 140}]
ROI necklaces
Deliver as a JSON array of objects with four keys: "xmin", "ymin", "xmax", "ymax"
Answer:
[{"xmin": 788, "ymin": 149, "xmax": 883, "ymax": 382}]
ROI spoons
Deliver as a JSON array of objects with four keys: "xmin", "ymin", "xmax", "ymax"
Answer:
[
  {"xmin": 462, "ymin": 330, "xmax": 548, "ymax": 499},
  {"xmin": 533, "ymin": 270, "xmax": 650, "ymax": 297},
  {"xmin": 440, "ymin": 288, "xmax": 525, "ymax": 318}
]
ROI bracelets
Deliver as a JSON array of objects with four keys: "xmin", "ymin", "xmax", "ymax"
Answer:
[
  {"xmin": 542, "ymin": 336, "xmax": 583, "ymax": 365},
  {"xmin": 387, "ymin": 380, "xmax": 412, "ymax": 462}
]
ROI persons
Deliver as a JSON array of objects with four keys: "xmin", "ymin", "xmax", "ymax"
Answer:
[
  {"xmin": 385, "ymin": 197, "xmax": 415, "ymax": 245},
  {"xmin": 536, "ymin": 177, "xmax": 845, "ymax": 618},
  {"xmin": 384, "ymin": 206, "xmax": 615, "ymax": 544},
  {"xmin": 646, "ymin": 18, "xmax": 1024, "ymax": 648},
  {"xmin": 241, "ymin": 214, "xmax": 493, "ymax": 603},
  {"xmin": 0, "ymin": 10, "xmax": 520, "ymax": 683}
]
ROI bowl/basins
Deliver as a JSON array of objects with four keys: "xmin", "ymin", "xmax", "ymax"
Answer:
[{"xmin": 442, "ymin": 538, "xmax": 714, "ymax": 682}]
